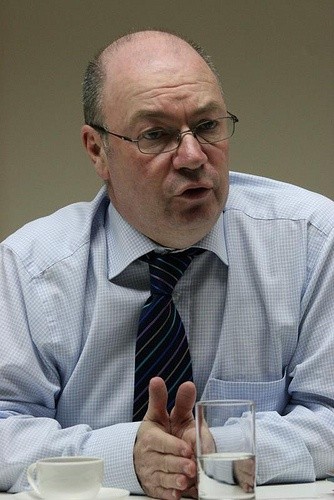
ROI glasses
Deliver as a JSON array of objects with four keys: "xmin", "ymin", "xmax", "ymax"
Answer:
[{"xmin": 90, "ymin": 110, "xmax": 240, "ymax": 154}]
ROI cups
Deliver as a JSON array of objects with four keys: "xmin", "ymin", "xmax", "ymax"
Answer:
[
  {"xmin": 27, "ymin": 457, "xmax": 103, "ymax": 500},
  {"xmin": 196, "ymin": 400, "xmax": 256, "ymax": 500}
]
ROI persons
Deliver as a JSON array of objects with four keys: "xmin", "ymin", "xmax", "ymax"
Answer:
[{"xmin": 0, "ymin": 27, "xmax": 334, "ymax": 499}]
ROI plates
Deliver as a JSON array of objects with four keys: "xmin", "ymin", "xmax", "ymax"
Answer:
[{"xmin": 12, "ymin": 486, "xmax": 129, "ymax": 500}]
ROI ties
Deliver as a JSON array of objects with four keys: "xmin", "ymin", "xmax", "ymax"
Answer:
[{"xmin": 130, "ymin": 246, "xmax": 209, "ymax": 425}]
